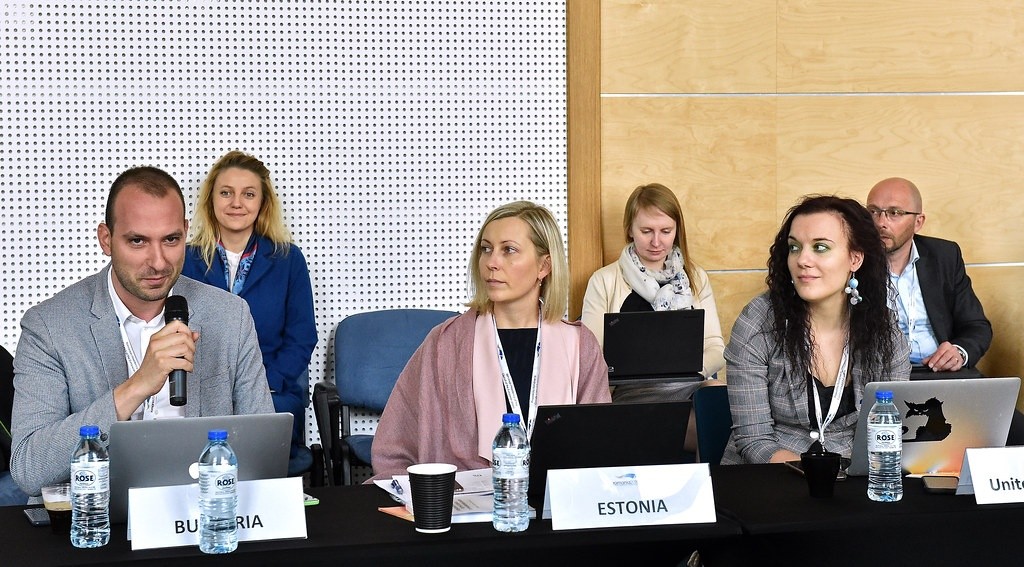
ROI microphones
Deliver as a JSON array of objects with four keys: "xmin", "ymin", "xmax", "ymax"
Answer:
[{"xmin": 163, "ymin": 295, "xmax": 189, "ymax": 406}]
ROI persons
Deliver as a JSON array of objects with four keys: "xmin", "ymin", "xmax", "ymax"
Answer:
[
  {"xmin": 866, "ymin": 178, "xmax": 993, "ymax": 372},
  {"xmin": 581, "ymin": 183, "xmax": 726, "ymax": 451},
  {"xmin": 362, "ymin": 201, "xmax": 612, "ymax": 484},
  {"xmin": 180, "ymin": 150, "xmax": 319, "ymax": 460},
  {"xmin": 720, "ymin": 196, "xmax": 912, "ymax": 465},
  {"xmin": 9, "ymin": 167, "xmax": 276, "ymax": 506}
]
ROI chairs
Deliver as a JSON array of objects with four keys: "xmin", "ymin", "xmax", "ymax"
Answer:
[
  {"xmin": 316, "ymin": 308, "xmax": 461, "ymax": 487},
  {"xmin": 693, "ymin": 385, "xmax": 732, "ymax": 469}
]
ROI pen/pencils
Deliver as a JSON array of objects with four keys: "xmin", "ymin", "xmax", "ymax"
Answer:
[{"xmin": 391, "ymin": 479, "xmax": 403, "ymax": 494}]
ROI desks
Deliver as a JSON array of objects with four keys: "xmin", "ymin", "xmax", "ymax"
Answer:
[{"xmin": 0, "ymin": 452, "xmax": 1024, "ymax": 567}]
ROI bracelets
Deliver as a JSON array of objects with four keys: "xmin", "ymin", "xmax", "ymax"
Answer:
[{"xmin": 958, "ymin": 348, "xmax": 966, "ymax": 362}]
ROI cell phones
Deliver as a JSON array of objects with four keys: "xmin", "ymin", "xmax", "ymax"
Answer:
[
  {"xmin": 922, "ymin": 476, "xmax": 960, "ymax": 493},
  {"xmin": 24, "ymin": 507, "xmax": 51, "ymax": 526},
  {"xmin": 785, "ymin": 461, "xmax": 847, "ymax": 481},
  {"xmin": 455, "ymin": 480, "xmax": 463, "ymax": 492}
]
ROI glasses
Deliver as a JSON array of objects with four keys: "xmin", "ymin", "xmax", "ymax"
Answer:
[{"xmin": 868, "ymin": 206, "xmax": 922, "ymax": 222}]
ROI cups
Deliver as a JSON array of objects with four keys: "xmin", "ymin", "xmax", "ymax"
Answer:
[
  {"xmin": 407, "ymin": 462, "xmax": 458, "ymax": 533},
  {"xmin": 800, "ymin": 451, "xmax": 841, "ymax": 501}
]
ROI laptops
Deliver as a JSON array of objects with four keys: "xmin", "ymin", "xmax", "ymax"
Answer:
[
  {"xmin": 603, "ymin": 309, "xmax": 706, "ymax": 386},
  {"xmin": 842, "ymin": 377, "xmax": 1021, "ymax": 474},
  {"xmin": 527, "ymin": 400, "xmax": 692, "ymax": 509},
  {"xmin": 109, "ymin": 413, "xmax": 294, "ymax": 526}
]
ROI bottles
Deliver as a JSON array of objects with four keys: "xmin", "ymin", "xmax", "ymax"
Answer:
[
  {"xmin": 198, "ymin": 429, "xmax": 238, "ymax": 554},
  {"xmin": 70, "ymin": 425, "xmax": 110, "ymax": 548},
  {"xmin": 492, "ymin": 413, "xmax": 530, "ymax": 533},
  {"xmin": 866, "ymin": 389, "xmax": 903, "ymax": 502}
]
